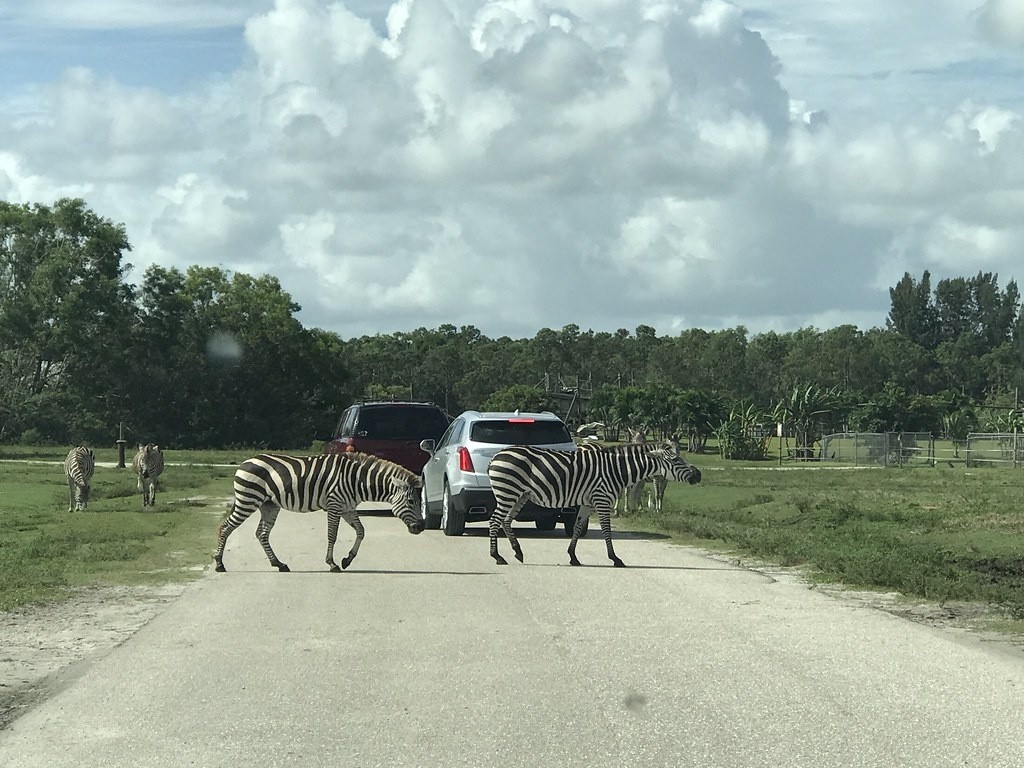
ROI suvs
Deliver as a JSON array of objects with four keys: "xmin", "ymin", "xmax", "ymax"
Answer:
[{"xmin": 418, "ymin": 408, "xmax": 588, "ymax": 538}]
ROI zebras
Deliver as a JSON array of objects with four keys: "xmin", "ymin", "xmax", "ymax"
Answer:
[
  {"xmin": 213, "ymin": 451, "xmax": 426, "ymax": 573},
  {"xmin": 133, "ymin": 443, "xmax": 165, "ymax": 507},
  {"xmin": 64, "ymin": 447, "xmax": 95, "ymax": 513},
  {"xmin": 487, "ymin": 424, "xmax": 702, "ymax": 568}
]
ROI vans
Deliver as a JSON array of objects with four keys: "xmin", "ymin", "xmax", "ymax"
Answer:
[{"xmin": 326, "ymin": 399, "xmax": 450, "ymax": 476}]
